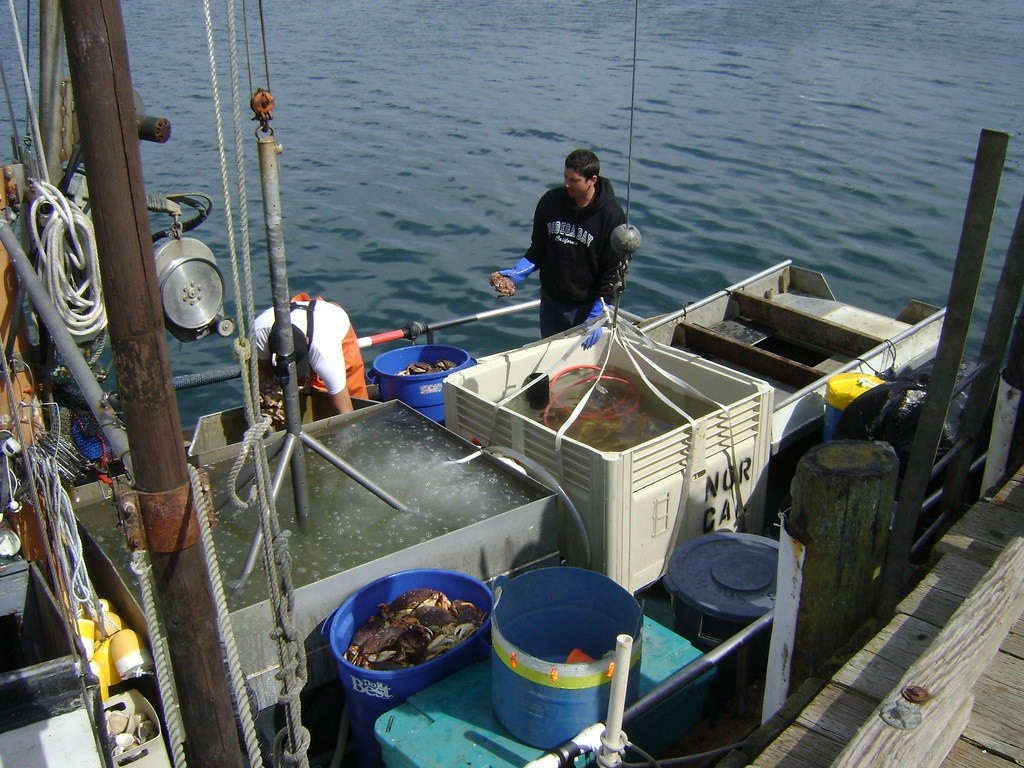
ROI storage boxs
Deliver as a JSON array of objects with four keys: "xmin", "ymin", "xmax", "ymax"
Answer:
[
  {"xmin": 373, "ymin": 613, "xmax": 720, "ymax": 768},
  {"xmin": 443, "ymin": 327, "xmax": 775, "ymax": 596},
  {"xmin": 187, "ymin": 389, "xmax": 383, "ymax": 456}
]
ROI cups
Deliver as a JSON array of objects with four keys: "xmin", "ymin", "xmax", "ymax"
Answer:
[{"xmin": 527, "ymin": 373, "xmax": 549, "ymax": 410}]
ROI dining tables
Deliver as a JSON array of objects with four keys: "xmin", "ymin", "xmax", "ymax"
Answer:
[{"xmin": 103, "ymin": 689, "xmax": 171, "ymax": 768}]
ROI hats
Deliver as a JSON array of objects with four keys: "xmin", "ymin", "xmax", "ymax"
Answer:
[{"xmin": 268, "ymin": 324, "xmax": 309, "ymax": 383}]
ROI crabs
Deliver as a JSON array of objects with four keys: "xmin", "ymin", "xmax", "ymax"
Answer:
[
  {"xmin": 395, "ymin": 358, "xmax": 456, "ymax": 377},
  {"xmin": 342, "ymin": 588, "xmax": 489, "ymax": 666}
]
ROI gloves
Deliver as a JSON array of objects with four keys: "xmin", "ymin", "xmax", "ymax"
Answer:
[
  {"xmin": 490, "ymin": 258, "xmax": 537, "ymax": 298},
  {"xmin": 581, "ymin": 300, "xmax": 611, "ymax": 349}
]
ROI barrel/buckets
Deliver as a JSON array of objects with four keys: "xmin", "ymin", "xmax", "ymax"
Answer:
[
  {"xmin": 663, "ymin": 532, "xmax": 780, "ymax": 688},
  {"xmin": 365, "ymin": 345, "xmax": 477, "ymax": 427},
  {"xmin": 321, "ymin": 567, "xmax": 492, "ymax": 768},
  {"xmin": 823, "ymin": 372, "xmax": 886, "ymax": 440},
  {"xmin": 493, "ymin": 568, "xmax": 645, "ymax": 750}
]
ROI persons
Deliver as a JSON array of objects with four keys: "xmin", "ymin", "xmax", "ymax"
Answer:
[
  {"xmin": 255, "ymin": 291, "xmax": 368, "ymax": 416},
  {"xmin": 499, "ymin": 149, "xmax": 628, "ymax": 350}
]
